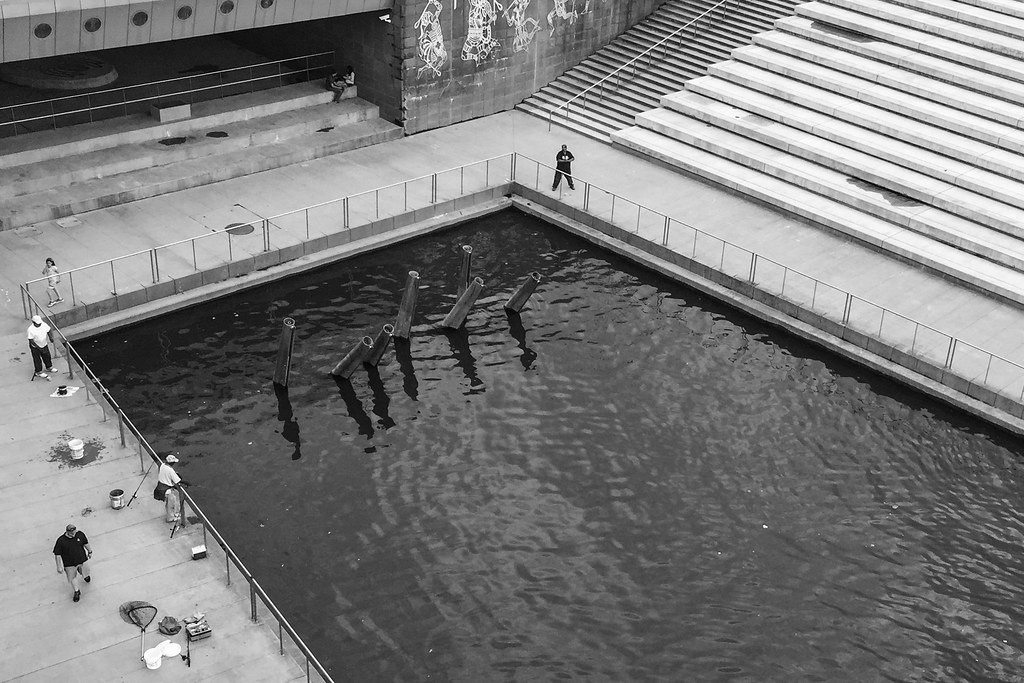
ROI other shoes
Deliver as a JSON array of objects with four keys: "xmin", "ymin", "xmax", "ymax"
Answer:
[
  {"xmin": 56, "ymin": 298, "xmax": 64, "ymax": 302},
  {"xmin": 73, "ymin": 590, "xmax": 80, "ymax": 602},
  {"xmin": 84, "ymin": 576, "xmax": 90, "ymax": 583},
  {"xmin": 36, "ymin": 372, "xmax": 48, "ymax": 378},
  {"xmin": 47, "ymin": 301, "xmax": 56, "ymax": 307},
  {"xmin": 46, "ymin": 367, "xmax": 58, "ymax": 373}
]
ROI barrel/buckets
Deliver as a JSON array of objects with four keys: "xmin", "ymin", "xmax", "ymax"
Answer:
[
  {"xmin": 109, "ymin": 489, "xmax": 125, "ymax": 510},
  {"xmin": 69, "ymin": 439, "xmax": 84, "ymax": 460}
]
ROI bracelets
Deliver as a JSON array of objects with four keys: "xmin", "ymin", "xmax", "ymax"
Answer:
[{"xmin": 88, "ymin": 551, "xmax": 92, "ymax": 554}]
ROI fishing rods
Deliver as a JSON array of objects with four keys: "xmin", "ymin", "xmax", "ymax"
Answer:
[
  {"xmin": 169, "ymin": 493, "xmax": 186, "ymax": 538},
  {"xmin": 126, "ymin": 460, "xmax": 155, "ymax": 507}
]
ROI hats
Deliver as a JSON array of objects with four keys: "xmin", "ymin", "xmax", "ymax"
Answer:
[
  {"xmin": 32, "ymin": 315, "xmax": 42, "ymax": 325},
  {"xmin": 166, "ymin": 455, "xmax": 179, "ymax": 463}
]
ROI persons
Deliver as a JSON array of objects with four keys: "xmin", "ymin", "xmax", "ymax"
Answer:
[
  {"xmin": 326, "ymin": 71, "xmax": 344, "ymax": 104},
  {"xmin": 336, "ymin": 65, "xmax": 355, "ymax": 88},
  {"xmin": 53, "ymin": 524, "xmax": 92, "ymax": 602},
  {"xmin": 552, "ymin": 144, "xmax": 575, "ymax": 191},
  {"xmin": 154, "ymin": 454, "xmax": 192, "ymax": 522},
  {"xmin": 27, "ymin": 315, "xmax": 58, "ymax": 378},
  {"xmin": 42, "ymin": 258, "xmax": 65, "ymax": 307}
]
